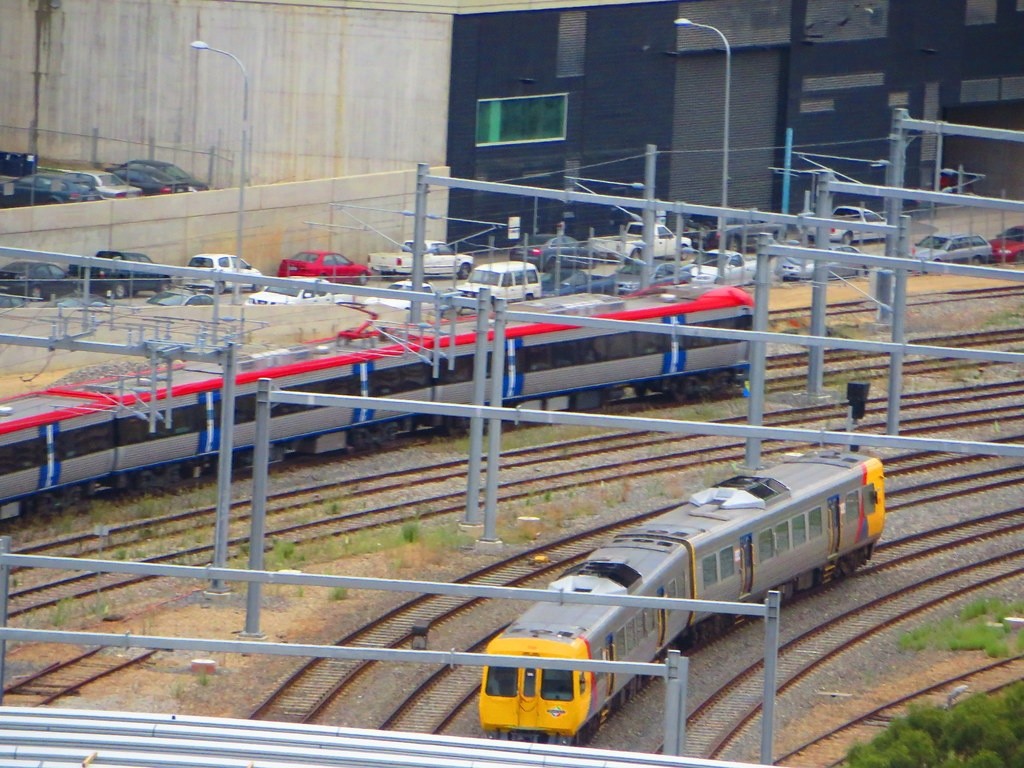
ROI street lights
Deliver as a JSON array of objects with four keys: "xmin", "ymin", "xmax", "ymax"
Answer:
[
  {"xmin": 674, "ymin": 18, "xmax": 730, "ymax": 286},
  {"xmin": 191, "ymin": 41, "xmax": 248, "ymax": 305}
]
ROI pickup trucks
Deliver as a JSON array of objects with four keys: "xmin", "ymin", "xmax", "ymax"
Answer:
[
  {"xmin": 807, "ymin": 206, "xmax": 889, "ymax": 244},
  {"xmin": 684, "ymin": 248, "xmax": 759, "ymax": 287},
  {"xmin": 69, "ymin": 251, "xmax": 172, "ymax": 298},
  {"xmin": 587, "ymin": 222, "xmax": 693, "ymax": 262},
  {"xmin": 366, "ymin": 240, "xmax": 475, "ymax": 282}
]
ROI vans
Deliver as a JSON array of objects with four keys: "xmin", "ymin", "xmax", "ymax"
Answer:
[{"xmin": 462, "ymin": 261, "xmax": 542, "ymax": 310}]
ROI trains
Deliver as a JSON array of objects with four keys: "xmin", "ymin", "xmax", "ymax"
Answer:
[
  {"xmin": 1, "ymin": 284, "xmax": 756, "ymax": 520},
  {"xmin": 480, "ymin": 448, "xmax": 887, "ymax": 748}
]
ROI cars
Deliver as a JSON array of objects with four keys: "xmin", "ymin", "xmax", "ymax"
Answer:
[
  {"xmin": 366, "ymin": 281, "xmax": 434, "ymax": 311},
  {"xmin": 900, "ymin": 165, "xmax": 974, "ymax": 207},
  {"xmin": 0, "ymin": 151, "xmax": 209, "ymax": 207},
  {"xmin": 510, "ymin": 234, "xmax": 598, "ymax": 272},
  {"xmin": 604, "ymin": 261, "xmax": 692, "ymax": 296},
  {"xmin": 716, "ymin": 223, "xmax": 786, "ymax": 253},
  {"xmin": 908, "ymin": 232, "xmax": 994, "ymax": 274},
  {"xmin": 683, "ymin": 207, "xmax": 786, "ymax": 254},
  {"xmin": 239, "ymin": 278, "xmax": 354, "ymax": 307},
  {"xmin": 0, "ymin": 260, "xmax": 78, "ymax": 300},
  {"xmin": 278, "ymin": 250, "xmax": 371, "ymax": 286},
  {"xmin": 774, "ymin": 245, "xmax": 870, "ymax": 282},
  {"xmin": 987, "ymin": 225, "xmax": 1024, "ymax": 263},
  {"xmin": 144, "ymin": 291, "xmax": 214, "ymax": 306},
  {"xmin": 183, "ymin": 254, "xmax": 264, "ymax": 294}
]
